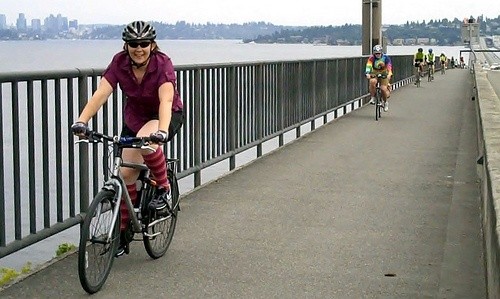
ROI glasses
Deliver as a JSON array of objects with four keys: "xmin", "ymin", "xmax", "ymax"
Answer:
[{"xmin": 127, "ymin": 41, "xmax": 151, "ymax": 49}]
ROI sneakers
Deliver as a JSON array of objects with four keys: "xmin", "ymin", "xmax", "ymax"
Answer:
[
  {"xmin": 147, "ymin": 186, "xmax": 172, "ymax": 210},
  {"xmin": 117, "ymin": 230, "xmax": 134, "ymax": 256}
]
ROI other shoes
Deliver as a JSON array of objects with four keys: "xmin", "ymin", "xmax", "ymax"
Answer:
[
  {"xmin": 369, "ymin": 96, "xmax": 375, "ymax": 105},
  {"xmin": 383, "ymin": 102, "xmax": 389, "ymax": 112}
]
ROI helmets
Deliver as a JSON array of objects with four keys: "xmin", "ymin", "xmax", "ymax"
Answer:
[
  {"xmin": 418, "ymin": 48, "xmax": 423, "ymax": 52},
  {"xmin": 122, "ymin": 21, "xmax": 157, "ymax": 42},
  {"xmin": 373, "ymin": 45, "xmax": 384, "ymax": 54},
  {"xmin": 428, "ymin": 49, "xmax": 432, "ymax": 52}
]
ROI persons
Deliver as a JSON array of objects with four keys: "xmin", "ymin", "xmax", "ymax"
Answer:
[
  {"xmin": 413, "ymin": 48, "xmax": 425, "ymax": 85},
  {"xmin": 426, "ymin": 49, "xmax": 436, "ymax": 80},
  {"xmin": 451, "ymin": 56, "xmax": 465, "ymax": 69},
  {"xmin": 71, "ymin": 20, "xmax": 186, "ymax": 258},
  {"xmin": 366, "ymin": 45, "xmax": 391, "ymax": 112},
  {"xmin": 439, "ymin": 53, "xmax": 446, "ymax": 72}
]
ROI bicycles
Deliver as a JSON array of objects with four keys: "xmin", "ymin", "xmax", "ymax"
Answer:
[
  {"xmin": 412, "ymin": 62, "xmax": 426, "ymax": 88},
  {"xmin": 364, "ymin": 74, "xmax": 387, "ymax": 121},
  {"xmin": 73, "ymin": 128, "xmax": 182, "ymax": 295},
  {"xmin": 427, "ymin": 62, "xmax": 434, "ymax": 83},
  {"xmin": 440, "ymin": 62, "xmax": 446, "ymax": 75}
]
